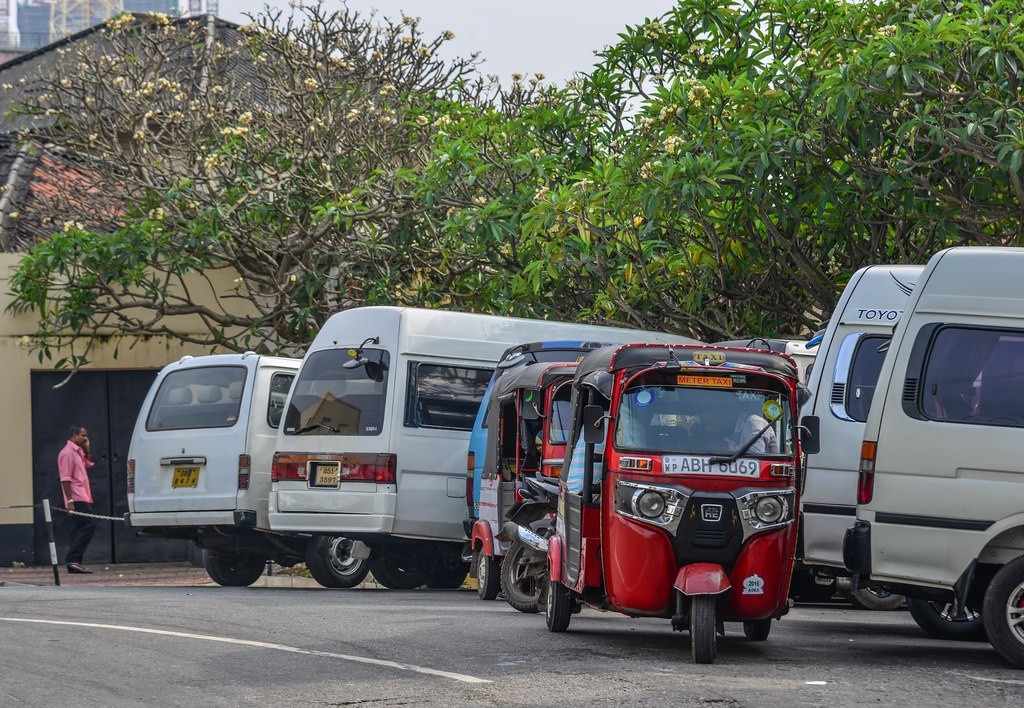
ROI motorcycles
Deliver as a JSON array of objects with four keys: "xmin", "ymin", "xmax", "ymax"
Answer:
[{"xmin": 461, "ymin": 342, "xmax": 823, "ymax": 664}]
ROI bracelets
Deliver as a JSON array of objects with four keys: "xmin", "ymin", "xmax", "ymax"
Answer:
[
  {"xmin": 85, "ymin": 450, "xmax": 92, "ymax": 454},
  {"xmin": 67, "ymin": 499, "xmax": 74, "ymax": 503}
]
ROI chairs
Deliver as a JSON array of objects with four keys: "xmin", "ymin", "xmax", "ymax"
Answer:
[
  {"xmin": 166, "ymin": 382, "xmax": 244, "ymax": 404},
  {"xmin": 270, "ymin": 396, "xmax": 284, "ymax": 412}
]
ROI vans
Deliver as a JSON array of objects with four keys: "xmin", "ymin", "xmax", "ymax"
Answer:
[
  {"xmin": 265, "ymin": 305, "xmax": 710, "ymax": 588},
  {"xmin": 121, "ymin": 349, "xmax": 427, "ymax": 588},
  {"xmin": 712, "ymin": 246, "xmax": 1024, "ymax": 671}
]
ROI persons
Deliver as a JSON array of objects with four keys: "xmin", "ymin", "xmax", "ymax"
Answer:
[
  {"xmin": 57, "ymin": 423, "xmax": 97, "ymax": 573},
  {"xmin": 622, "ymin": 401, "xmax": 655, "ymax": 450},
  {"xmin": 688, "ymin": 399, "xmax": 779, "ymax": 454}
]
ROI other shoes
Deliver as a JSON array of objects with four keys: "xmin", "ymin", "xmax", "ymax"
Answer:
[
  {"xmin": 67, "ymin": 567, "xmax": 93, "ymax": 573},
  {"xmin": 69, "ymin": 560, "xmax": 86, "ymax": 573}
]
